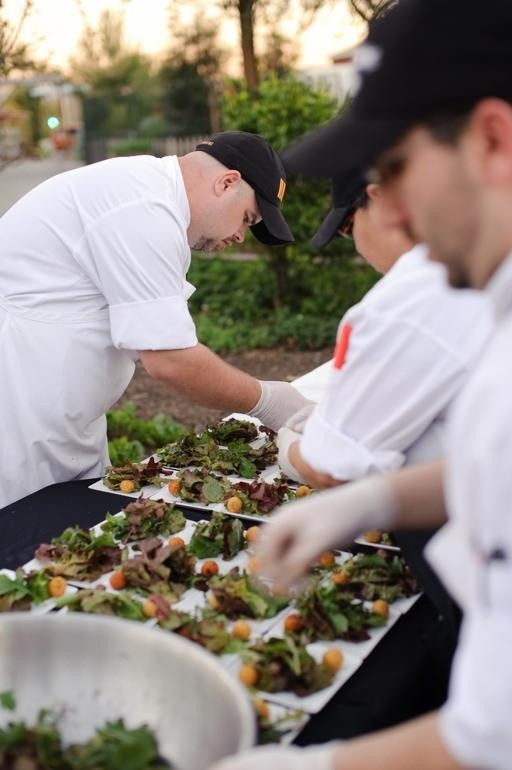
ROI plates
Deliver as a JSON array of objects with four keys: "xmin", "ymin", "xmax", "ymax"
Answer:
[
  {"xmin": 174, "ymin": 563, "xmax": 293, "ymax": 638},
  {"xmin": 87, "ymin": 462, "xmax": 179, "ymax": 500},
  {"xmin": 225, "ymin": 437, "xmax": 301, "ymax": 490},
  {"xmin": 169, "ymin": 518, "xmax": 251, "ymax": 576},
  {"xmin": 18, "ymin": 528, "xmax": 142, "ymax": 592},
  {"xmin": 269, "ymin": 587, "xmax": 400, "ymax": 659},
  {"xmin": 196, "ymin": 413, "xmax": 272, "ymax": 451},
  {"xmin": 147, "ymin": 466, "xmax": 250, "ymax": 510},
  {"xmin": 236, "ymin": 547, "xmax": 353, "ymax": 582},
  {"xmin": 258, "ymin": 700, "xmax": 309, "ymax": 742},
  {"xmin": 210, "ymin": 480, "xmax": 297, "ymax": 523},
  {"xmin": 309, "ymin": 488, "xmax": 405, "ymax": 549},
  {"xmin": 140, "ymin": 441, "xmax": 239, "ymax": 479},
  {"xmin": 92, "ymin": 509, "xmax": 198, "ymax": 548},
  {"xmin": 321, "ymin": 553, "xmax": 422, "ymax": 614},
  {"xmin": 99, "ymin": 558, "xmax": 205, "ymax": 608},
  {"xmin": 227, "ymin": 632, "xmax": 363, "ymax": 714},
  {"xmin": 0, "ymin": 567, "xmax": 80, "ymax": 611},
  {"xmin": 146, "ymin": 598, "xmax": 258, "ymax": 669}
]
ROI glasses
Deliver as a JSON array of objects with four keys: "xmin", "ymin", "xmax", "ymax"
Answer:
[{"xmin": 336, "ymin": 214, "xmax": 355, "ymax": 240}]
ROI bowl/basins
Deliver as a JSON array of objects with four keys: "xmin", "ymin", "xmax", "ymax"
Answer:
[{"xmin": 0, "ymin": 612, "xmax": 256, "ymax": 768}]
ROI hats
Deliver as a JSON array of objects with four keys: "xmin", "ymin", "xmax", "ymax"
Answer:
[
  {"xmin": 198, "ymin": 131, "xmax": 294, "ymax": 246},
  {"xmin": 283, "ymin": 1, "xmax": 511, "ymax": 177},
  {"xmin": 302, "ymin": 166, "xmax": 368, "ymax": 248}
]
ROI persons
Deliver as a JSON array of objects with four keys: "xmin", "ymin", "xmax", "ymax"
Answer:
[
  {"xmin": 275, "ymin": 172, "xmax": 501, "ymax": 729},
  {"xmin": 207, "ymin": 2, "xmax": 512, "ymax": 769},
  {"xmin": 0, "ymin": 125, "xmax": 312, "ymax": 513}
]
